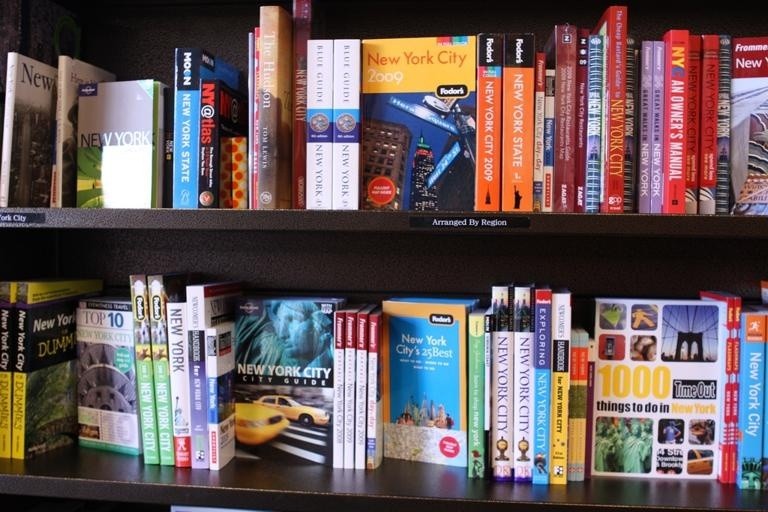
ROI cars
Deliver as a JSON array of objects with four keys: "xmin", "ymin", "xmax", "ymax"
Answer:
[{"xmin": 233, "ymin": 393, "xmax": 331, "ymax": 447}]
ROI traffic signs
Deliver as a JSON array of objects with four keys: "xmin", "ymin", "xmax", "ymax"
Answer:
[{"xmin": 390, "ymin": 95, "xmax": 477, "ymax": 211}]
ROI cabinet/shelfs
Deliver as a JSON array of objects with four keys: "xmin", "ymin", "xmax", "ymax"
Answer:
[{"xmin": 0, "ymin": 1, "xmax": 767, "ymax": 511}]
